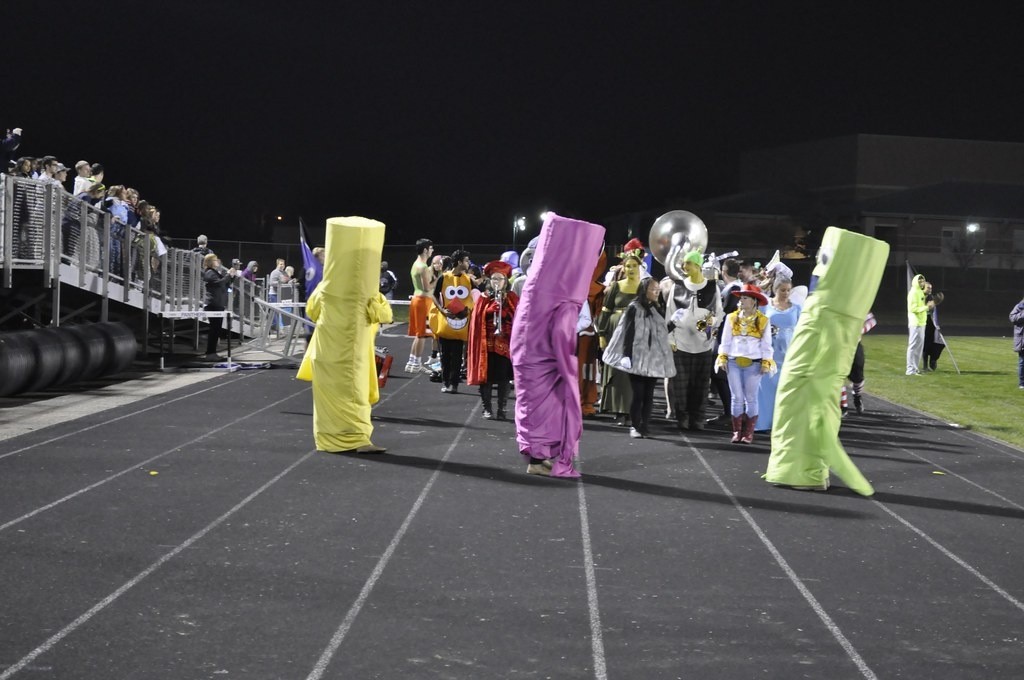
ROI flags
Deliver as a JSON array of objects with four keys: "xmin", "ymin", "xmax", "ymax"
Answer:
[
  {"xmin": 907, "ymin": 263, "xmax": 946, "ymax": 360},
  {"xmin": 300, "ymin": 231, "xmax": 323, "ymax": 331}
]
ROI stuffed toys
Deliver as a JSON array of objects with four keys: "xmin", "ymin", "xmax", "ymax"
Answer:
[{"xmin": 512, "ymin": 247, "xmax": 537, "ymax": 296}]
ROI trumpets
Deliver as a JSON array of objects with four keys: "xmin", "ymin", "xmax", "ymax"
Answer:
[
  {"xmin": 492, "ymin": 284, "xmax": 507, "ymax": 336},
  {"xmin": 696, "ymin": 310, "xmax": 714, "ymax": 332}
]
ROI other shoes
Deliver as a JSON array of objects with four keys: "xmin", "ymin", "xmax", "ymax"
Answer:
[
  {"xmin": 853, "ymin": 394, "xmax": 864, "ymax": 414},
  {"xmin": 840, "ymin": 408, "xmax": 848, "ymax": 418},
  {"xmin": 205, "ymin": 353, "xmax": 223, "ymax": 362},
  {"xmin": 906, "ymin": 368, "xmax": 921, "ymax": 376},
  {"xmin": 482, "ymin": 410, "xmax": 493, "ymax": 420},
  {"xmin": 357, "ymin": 443, "xmax": 387, "ymax": 453},
  {"xmin": 497, "ymin": 409, "xmax": 506, "ymax": 420},
  {"xmin": 423, "ymin": 356, "xmax": 437, "ymax": 364},
  {"xmin": 583, "ymin": 397, "xmax": 732, "ymax": 438},
  {"xmin": 526, "ymin": 458, "xmax": 554, "ymax": 477},
  {"xmin": 440, "ymin": 381, "xmax": 456, "ymax": 393}
]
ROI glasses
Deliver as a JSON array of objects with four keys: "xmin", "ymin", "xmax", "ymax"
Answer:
[{"xmin": 491, "ymin": 276, "xmax": 503, "ymax": 280}]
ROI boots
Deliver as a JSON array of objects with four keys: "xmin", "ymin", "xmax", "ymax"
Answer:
[
  {"xmin": 730, "ymin": 412, "xmax": 745, "ymax": 443},
  {"xmin": 740, "ymin": 415, "xmax": 758, "ymax": 443}
]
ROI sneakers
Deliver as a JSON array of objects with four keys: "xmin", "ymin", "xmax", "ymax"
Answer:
[{"xmin": 404, "ymin": 360, "xmax": 432, "ymax": 375}]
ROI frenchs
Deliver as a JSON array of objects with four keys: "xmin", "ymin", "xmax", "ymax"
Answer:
[{"xmin": 648, "ymin": 209, "xmax": 722, "ymax": 282}]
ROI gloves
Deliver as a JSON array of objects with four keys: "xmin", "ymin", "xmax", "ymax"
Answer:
[{"xmin": 621, "ymin": 357, "xmax": 631, "ymax": 369}]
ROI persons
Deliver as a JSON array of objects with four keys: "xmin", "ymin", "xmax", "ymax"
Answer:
[
  {"xmin": 0, "ymin": 127, "xmax": 169, "ymax": 293},
  {"xmin": 202, "ymin": 254, "xmax": 236, "ymax": 361},
  {"xmin": 1009, "ymin": 299, "xmax": 1024, "ymax": 388},
  {"xmin": 232, "ymin": 258, "xmax": 243, "ymax": 314},
  {"xmin": 241, "ymin": 261, "xmax": 260, "ymax": 321},
  {"xmin": 379, "ymin": 262, "xmax": 397, "ymax": 301},
  {"xmin": 906, "ymin": 274, "xmax": 937, "ymax": 375},
  {"xmin": 268, "ymin": 259, "xmax": 297, "ymax": 334},
  {"xmin": 185, "ymin": 235, "xmax": 213, "ymax": 264},
  {"xmin": 405, "ymin": 238, "xmax": 875, "ymax": 444},
  {"xmin": 306, "ymin": 246, "xmax": 325, "ymax": 343}
]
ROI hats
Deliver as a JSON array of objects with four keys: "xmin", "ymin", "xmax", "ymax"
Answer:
[
  {"xmin": 731, "ymin": 285, "xmax": 768, "ymax": 306},
  {"xmin": 624, "ymin": 238, "xmax": 644, "ymax": 251},
  {"xmin": 501, "ymin": 251, "xmax": 518, "ymax": 267},
  {"xmin": 684, "ymin": 247, "xmax": 703, "ymax": 267},
  {"xmin": 232, "ymin": 259, "xmax": 243, "ymax": 265},
  {"xmin": 484, "ymin": 261, "xmax": 512, "ymax": 280},
  {"xmin": 52, "ymin": 163, "xmax": 71, "ymax": 174},
  {"xmin": 702, "ymin": 253, "xmax": 721, "ymax": 274}
]
